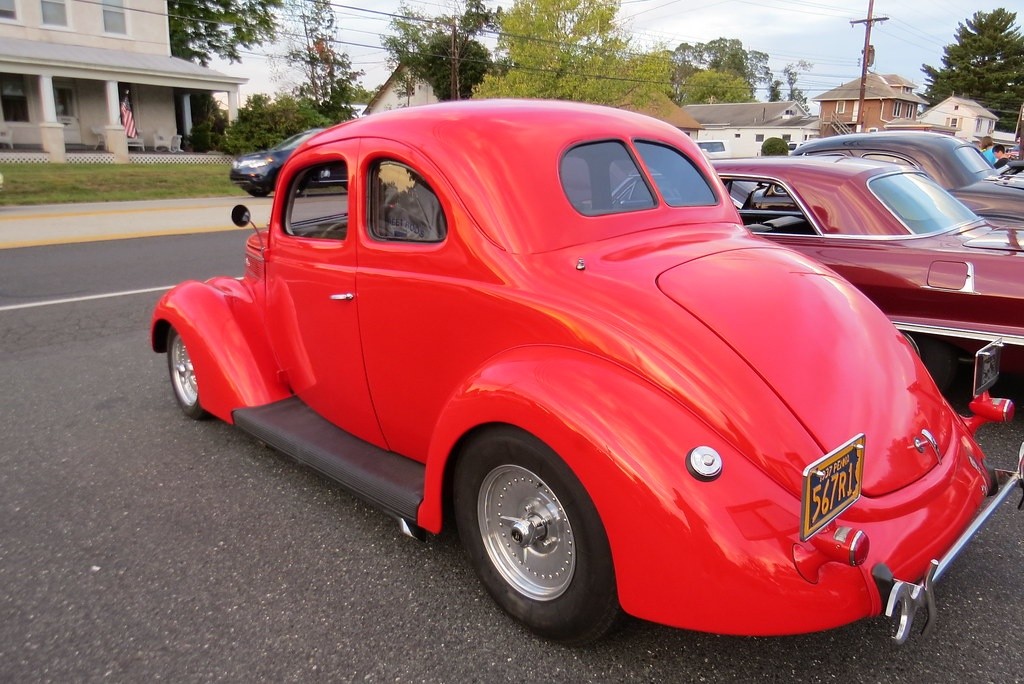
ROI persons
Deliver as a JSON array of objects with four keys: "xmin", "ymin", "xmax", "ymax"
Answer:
[
  {"xmin": 991, "ymin": 143, "xmax": 1009, "ymax": 169},
  {"xmin": 980, "ymin": 135, "xmax": 996, "ymax": 165},
  {"xmin": 380, "ymin": 173, "xmax": 452, "ymax": 242}
]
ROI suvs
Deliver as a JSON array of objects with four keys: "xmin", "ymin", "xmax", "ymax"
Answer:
[{"xmin": 230, "ymin": 129, "xmax": 348, "ymax": 197}]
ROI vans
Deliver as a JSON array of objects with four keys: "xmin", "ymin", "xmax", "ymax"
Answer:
[{"xmin": 695, "ymin": 141, "xmax": 729, "ymax": 160}]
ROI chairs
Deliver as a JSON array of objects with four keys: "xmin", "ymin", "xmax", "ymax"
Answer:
[
  {"xmin": 764, "ymin": 215, "xmax": 804, "ymax": 231},
  {"xmin": 0, "ymin": 123, "xmax": 15, "ymax": 150},
  {"xmin": 744, "ymin": 222, "xmax": 772, "ymax": 232},
  {"xmin": 153, "ymin": 128, "xmax": 174, "ymax": 151}
]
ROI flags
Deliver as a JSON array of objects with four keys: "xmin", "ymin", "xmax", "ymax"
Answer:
[{"xmin": 119, "ymin": 95, "xmax": 137, "ymax": 139}]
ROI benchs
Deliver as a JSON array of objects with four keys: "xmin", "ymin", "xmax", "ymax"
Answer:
[{"xmin": 88, "ymin": 126, "xmax": 145, "ymax": 151}]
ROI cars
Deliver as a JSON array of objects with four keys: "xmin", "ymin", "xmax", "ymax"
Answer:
[
  {"xmin": 789, "ymin": 131, "xmax": 1024, "ymax": 227},
  {"xmin": 708, "ymin": 156, "xmax": 1024, "ymax": 404},
  {"xmin": 150, "ymin": 98, "xmax": 1024, "ymax": 645}
]
